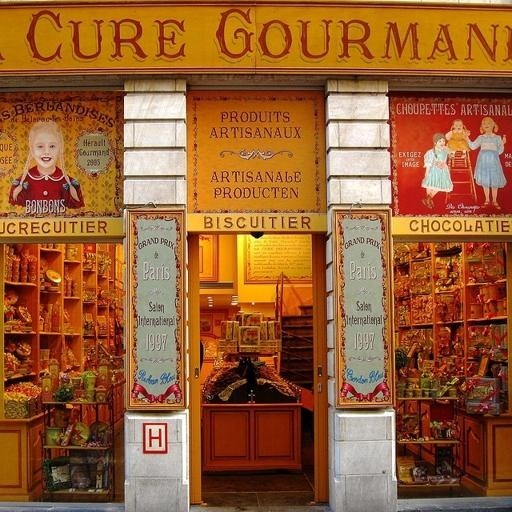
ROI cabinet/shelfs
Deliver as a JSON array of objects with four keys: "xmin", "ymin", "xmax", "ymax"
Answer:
[
  {"xmin": 0, "ymin": 409, "xmax": 48, "ymax": 503},
  {"xmin": 201, "ymin": 401, "xmax": 303, "ymax": 471},
  {"xmin": 396, "ymin": 399, "xmax": 452, "ymax": 466},
  {"xmin": 393, "ymin": 241, "xmax": 510, "ymax": 409},
  {"xmin": 453, "ymin": 411, "xmax": 512, "ymax": 497},
  {"xmin": 2, "ymin": 243, "xmax": 125, "ymax": 387},
  {"xmin": 281, "ymin": 314, "xmax": 313, "ymax": 384},
  {"xmin": 41, "ymin": 385, "xmax": 116, "ymax": 502}
]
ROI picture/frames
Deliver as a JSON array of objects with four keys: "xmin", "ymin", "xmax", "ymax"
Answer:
[
  {"xmin": 243, "ymin": 234, "xmax": 312, "ymax": 284},
  {"xmin": 198, "ymin": 234, "xmax": 220, "ymax": 283},
  {"xmin": 330, "ymin": 209, "xmax": 395, "ymax": 409},
  {"xmin": 124, "ymin": 205, "xmax": 187, "ymax": 411}
]
